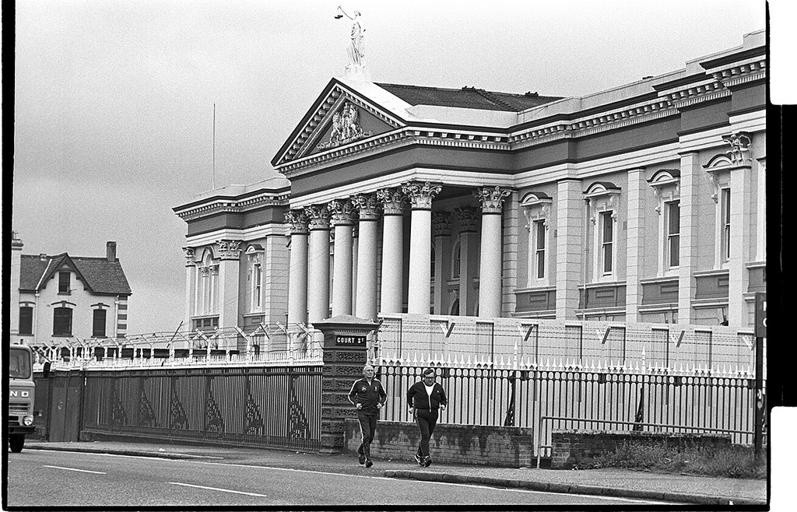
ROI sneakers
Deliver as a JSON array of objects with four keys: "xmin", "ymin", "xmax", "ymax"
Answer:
[
  {"xmin": 357, "ymin": 452, "xmax": 374, "ymax": 468},
  {"xmin": 414, "ymin": 454, "xmax": 432, "ymax": 467}
]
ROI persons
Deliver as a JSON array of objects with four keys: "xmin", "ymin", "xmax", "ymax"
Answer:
[
  {"xmin": 404, "ymin": 366, "xmax": 452, "ymax": 471},
  {"xmin": 345, "ymin": 362, "xmax": 387, "ymax": 470},
  {"xmin": 334, "ymin": 3, "xmax": 372, "ymax": 74}
]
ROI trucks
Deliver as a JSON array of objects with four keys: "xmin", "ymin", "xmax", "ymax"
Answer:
[{"xmin": 9, "ymin": 338, "xmax": 51, "ymax": 456}]
